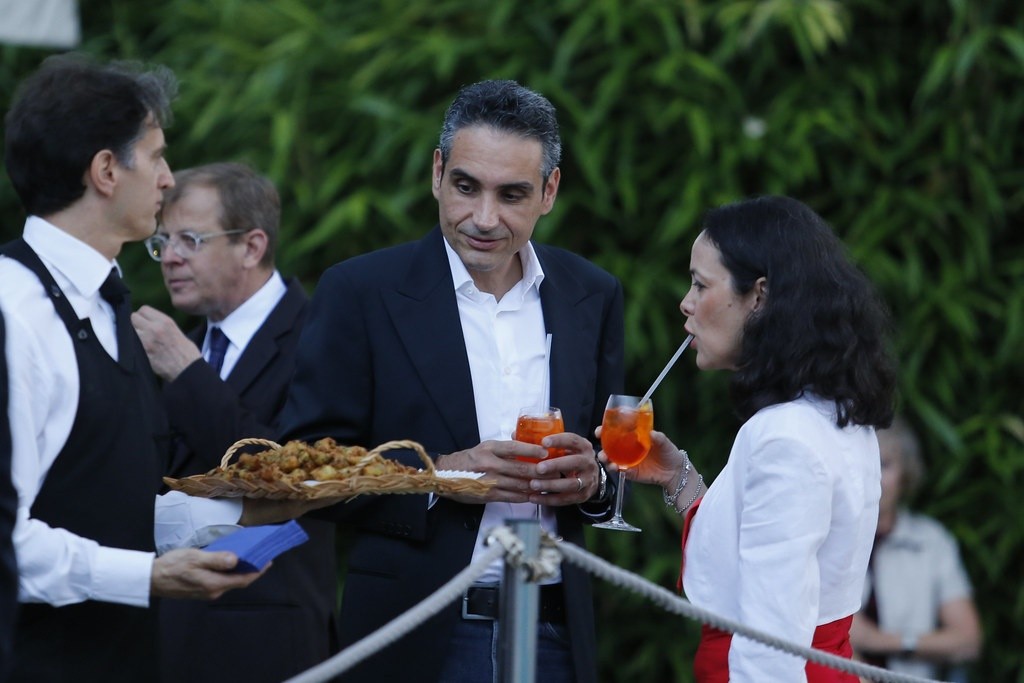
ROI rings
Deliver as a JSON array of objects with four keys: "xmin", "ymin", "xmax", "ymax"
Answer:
[{"xmin": 576, "ymin": 478, "xmax": 583, "ymax": 493}]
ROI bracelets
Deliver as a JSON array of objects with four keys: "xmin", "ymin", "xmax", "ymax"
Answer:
[
  {"xmin": 674, "ymin": 473, "xmax": 703, "ymax": 514},
  {"xmin": 663, "ymin": 449, "xmax": 692, "ymax": 508}
]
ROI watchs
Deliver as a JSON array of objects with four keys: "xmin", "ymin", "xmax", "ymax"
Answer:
[
  {"xmin": 595, "ymin": 457, "xmax": 608, "ymax": 502},
  {"xmin": 901, "ymin": 631, "xmax": 918, "ymax": 660}
]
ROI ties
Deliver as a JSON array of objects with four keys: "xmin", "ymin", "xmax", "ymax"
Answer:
[{"xmin": 862, "ymin": 538, "xmax": 890, "ymax": 670}]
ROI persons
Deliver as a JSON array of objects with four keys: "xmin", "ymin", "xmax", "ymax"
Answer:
[
  {"xmin": 593, "ymin": 194, "xmax": 902, "ymax": 683},
  {"xmin": 845, "ymin": 411, "xmax": 985, "ymax": 683},
  {"xmin": 275, "ymin": 78, "xmax": 629, "ymax": 683},
  {"xmin": 0, "ymin": 48, "xmax": 275, "ymax": 683},
  {"xmin": 129, "ymin": 160, "xmax": 337, "ymax": 682}
]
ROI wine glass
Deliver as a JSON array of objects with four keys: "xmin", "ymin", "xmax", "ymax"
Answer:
[
  {"xmin": 589, "ymin": 393, "xmax": 653, "ymax": 533},
  {"xmin": 515, "ymin": 404, "xmax": 565, "ymax": 547}
]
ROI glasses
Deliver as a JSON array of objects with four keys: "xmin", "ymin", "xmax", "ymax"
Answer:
[{"xmin": 145, "ymin": 229, "xmax": 252, "ymax": 263}]
